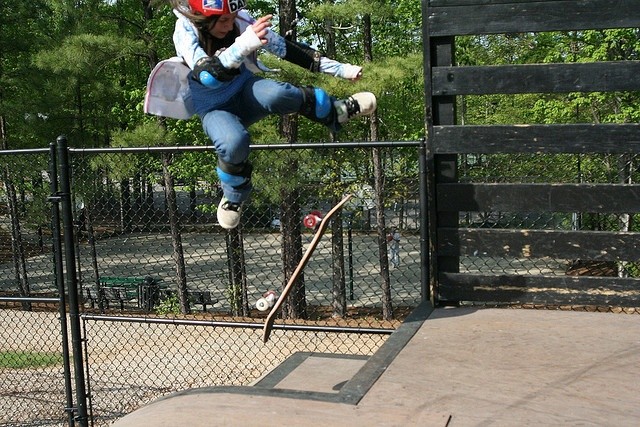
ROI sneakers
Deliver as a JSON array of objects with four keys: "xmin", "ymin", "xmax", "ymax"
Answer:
[
  {"xmin": 334, "ymin": 92, "xmax": 377, "ymax": 123},
  {"xmin": 217, "ymin": 196, "xmax": 245, "ymax": 229}
]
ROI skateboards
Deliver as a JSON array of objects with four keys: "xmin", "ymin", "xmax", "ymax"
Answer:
[{"xmin": 255, "ymin": 193, "xmax": 355, "ymax": 343}]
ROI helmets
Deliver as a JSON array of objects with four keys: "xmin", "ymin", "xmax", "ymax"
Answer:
[{"xmin": 188, "ymin": 0, "xmax": 247, "ymax": 16}]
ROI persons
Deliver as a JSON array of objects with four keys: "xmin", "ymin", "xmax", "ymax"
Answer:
[
  {"xmin": 144, "ymin": 0, "xmax": 377, "ymax": 229},
  {"xmin": 388, "ymin": 227, "xmax": 400, "ymax": 267}
]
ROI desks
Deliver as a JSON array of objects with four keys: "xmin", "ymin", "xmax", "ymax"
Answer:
[{"xmin": 98, "ymin": 276, "xmax": 159, "ymax": 293}]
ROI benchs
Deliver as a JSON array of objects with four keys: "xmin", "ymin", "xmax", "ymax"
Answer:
[
  {"xmin": 166, "ymin": 290, "xmax": 219, "ymax": 313},
  {"xmin": 84, "ymin": 288, "xmax": 138, "ymax": 310}
]
